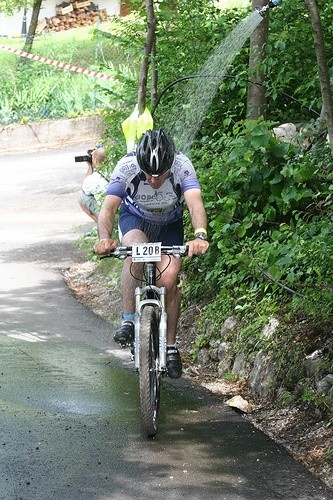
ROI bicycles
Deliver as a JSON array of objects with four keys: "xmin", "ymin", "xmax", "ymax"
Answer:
[{"xmin": 92, "ymin": 240, "xmax": 211, "ymax": 437}]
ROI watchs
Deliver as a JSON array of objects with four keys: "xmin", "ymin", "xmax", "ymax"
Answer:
[{"xmin": 196, "ymin": 233, "xmax": 207, "ymax": 239}]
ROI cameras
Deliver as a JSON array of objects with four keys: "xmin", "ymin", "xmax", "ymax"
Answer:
[{"xmin": 75, "ymin": 150, "xmax": 91, "ymax": 165}]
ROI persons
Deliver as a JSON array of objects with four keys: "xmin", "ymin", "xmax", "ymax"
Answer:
[
  {"xmin": 78, "ymin": 147, "xmax": 123, "ymax": 238},
  {"xmin": 93, "ymin": 128, "xmax": 210, "ymax": 379}
]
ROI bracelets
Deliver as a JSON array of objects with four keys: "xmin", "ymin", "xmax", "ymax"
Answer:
[{"xmin": 194, "ymin": 228, "xmax": 207, "ymax": 236}]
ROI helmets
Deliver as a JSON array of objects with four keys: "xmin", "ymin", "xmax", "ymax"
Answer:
[{"xmin": 136, "ymin": 128, "xmax": 175, "ymax": 177}]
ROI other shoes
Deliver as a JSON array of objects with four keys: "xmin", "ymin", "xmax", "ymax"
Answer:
[{"xmin": 85, "ymin": 227, "xmax": 97, "ymax": 240}]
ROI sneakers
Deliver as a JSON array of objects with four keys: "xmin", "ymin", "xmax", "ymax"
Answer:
[
  {"xmin": 166, "ymin": 346, "xmax": 182, "ymax": 378},
  {"xmin": 114, "ymin": 321, "xmax": 134, "ymax": 344}
]
